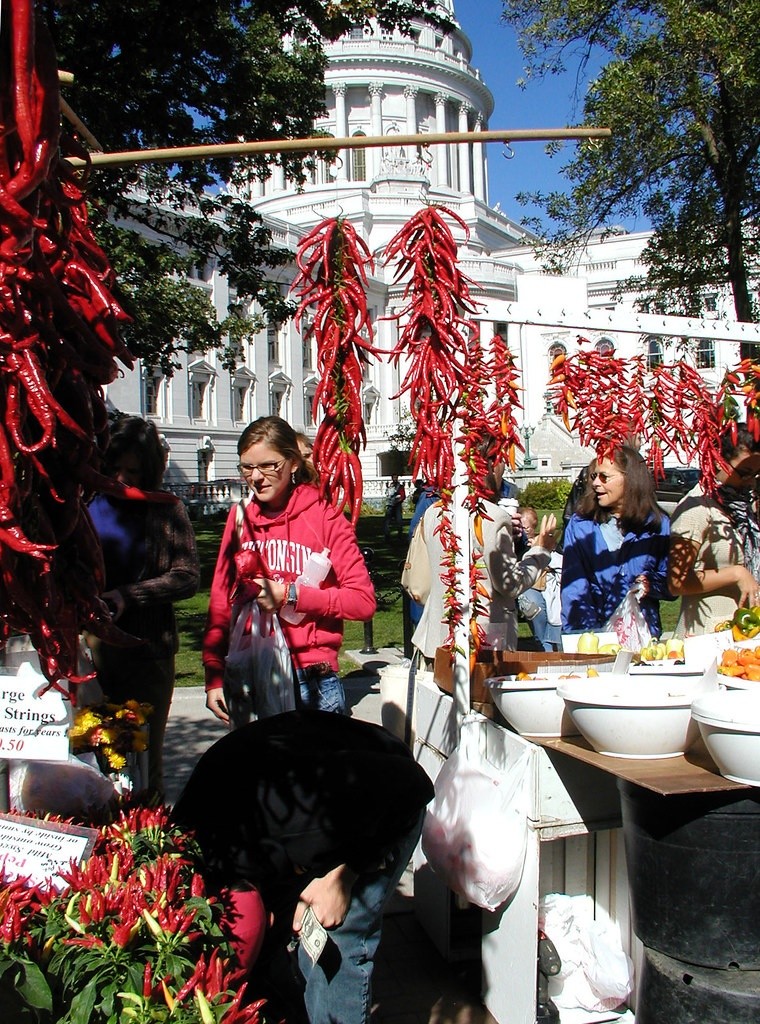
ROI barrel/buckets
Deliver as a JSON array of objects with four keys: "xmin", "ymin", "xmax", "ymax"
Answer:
[{"xmin": 615, "ymin": 770, "xmax": 760, "ymax": 972}]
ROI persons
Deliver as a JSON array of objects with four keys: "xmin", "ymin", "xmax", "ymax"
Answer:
[
  {"xmin": 667, "ymin": 422, "xmax": 760, "ymax": 641},
  {"xmin": 562, "ymin": 414, "xmax": 680, "ymax": 637},
  {"xmin": 78, "ymin": 415, "xmax": 198, "ymax": 799},
  {"xmin": 401, "ymin": 436, "xmax": 563, "ymax": 696},
  {"xmin": 164, "ymin": 711, "xmax": 436, "ymax": 1024}
]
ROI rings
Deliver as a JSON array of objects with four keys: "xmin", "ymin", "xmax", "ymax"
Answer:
[
  {"xmin": 549, "ymin": 533, "xmax": 554, "ymax": 537},
  {"xmin": 755, "ymin": 593, "xmax": 759, "ymax": 598}
]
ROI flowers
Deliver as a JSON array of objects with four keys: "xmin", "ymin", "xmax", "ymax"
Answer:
[{"xmin": 67, "ymin": 697, "xmax": 152, "ymax": 771}]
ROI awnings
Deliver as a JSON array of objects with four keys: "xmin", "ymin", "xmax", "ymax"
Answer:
[{"xmin": 202, "ymin": 415, "xmax": 376, "ymax": 732}]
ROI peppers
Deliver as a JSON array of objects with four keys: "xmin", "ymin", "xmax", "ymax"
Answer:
[
  {"xmin": 290, "ymin": 205, "xmax": 760, "ymax": 683},
  {"xmin": 0, "ymin": 2, "xmax": 267, "ymax": 1024}
]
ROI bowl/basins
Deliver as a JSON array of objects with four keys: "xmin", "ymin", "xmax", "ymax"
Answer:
[{"xmin": 483, "ymin": 653, "xmax": 759, "ymax": 788}]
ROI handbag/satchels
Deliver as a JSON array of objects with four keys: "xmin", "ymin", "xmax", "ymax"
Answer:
[
  {"xmin": 220, "ymin": 599, "xmax": 296, "ymax": 727},
  {"xmin": 401, "ymin": 515, "xmax": 431, "ymax": 607},
  {"xmin": 419, "ymin": 730, "xmax": 528, "ymax": 911},
  {"xmin": 603, "ymin": 583, "xmax": 652, "ymax": 653}
]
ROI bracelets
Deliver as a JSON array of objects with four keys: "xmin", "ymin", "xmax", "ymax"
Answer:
[{"xmin": 282, "ymin": 584, "xmax": 288, "ymax": 608}]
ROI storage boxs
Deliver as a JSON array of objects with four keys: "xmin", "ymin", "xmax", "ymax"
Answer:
[{"xmin": 433, "ymin": 646, "xmax": 644, "ymax": 702}]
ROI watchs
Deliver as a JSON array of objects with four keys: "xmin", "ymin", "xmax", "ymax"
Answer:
[{"xmin": 287, "ymin": 583, "xmax": 297, "ymax": 605}]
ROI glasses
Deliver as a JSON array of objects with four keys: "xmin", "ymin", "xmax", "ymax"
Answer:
[
  {"xmin": 590, "ymin": 472, "xmax": 627, "ymax": 484},
  {"xmin": 724, "ymin": 456, "xmax": 760, "ymax": 481},
  {"xmin": 237, "ymin": 456, "xmax": 291, "ymax": 477}
]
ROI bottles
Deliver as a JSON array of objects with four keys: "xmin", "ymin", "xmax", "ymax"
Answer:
[{"xmin": 280, "ymin": 547, "xmax": 332, "ymax": 624}]
